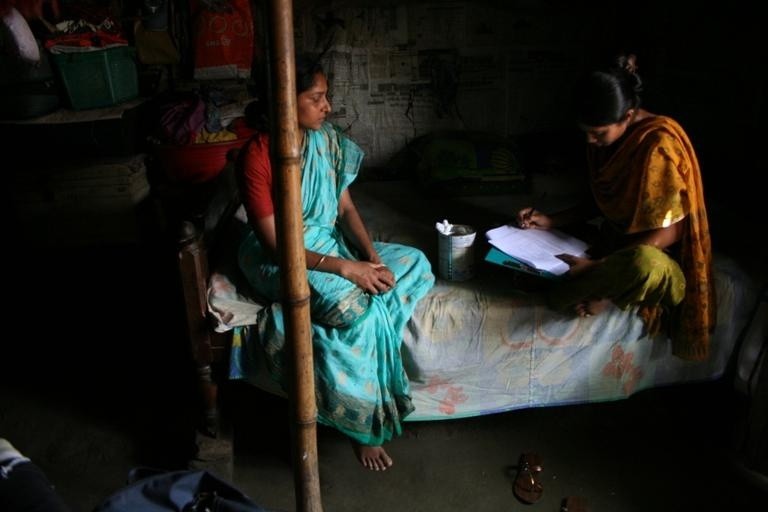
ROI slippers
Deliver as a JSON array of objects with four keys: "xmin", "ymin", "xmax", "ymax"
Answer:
[{"xmin": 513, "ymin": 452, "xmax": 544, "ymax": 504}]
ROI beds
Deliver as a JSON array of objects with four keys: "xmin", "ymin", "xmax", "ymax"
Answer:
[{"xmin": 172, "ymin": 145, "xmax": 768, "ymax": 443}]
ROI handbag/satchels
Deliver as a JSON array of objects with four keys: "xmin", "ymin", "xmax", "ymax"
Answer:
[{"xmin": 94, "ymin": 468, "xmax": 266, "ymax": 511}]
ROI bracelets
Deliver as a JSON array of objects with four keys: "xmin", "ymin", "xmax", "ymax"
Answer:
[{"xmin": 312, "ymin": 255, "xmax": 326, "ymax": 271}]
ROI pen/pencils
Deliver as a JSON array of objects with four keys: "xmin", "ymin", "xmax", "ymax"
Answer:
[{"xmin": 524, "ymin": 191, "xmax": 547, "ymax": 220}]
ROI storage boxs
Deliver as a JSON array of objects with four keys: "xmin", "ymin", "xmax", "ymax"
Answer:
[
  {"xmin": 49, "ymin": 151, "xmax": 149, "ymax": 215},
  {"xmin": 42, "ymin": 42, "xmax": 142, "ymax": 110}
]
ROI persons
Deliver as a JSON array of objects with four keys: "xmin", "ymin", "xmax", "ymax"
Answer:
[
  {"xmin": 513, "ymin": 54, "xmax": 716, "ymax": 362},
  {"xmin": 228, "ymin": 64, "xmax": 435, "ymax": 472}
]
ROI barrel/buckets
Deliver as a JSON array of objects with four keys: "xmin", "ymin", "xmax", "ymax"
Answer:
[{"xmin": 437, "ymin": 224, "xmax": 478, "ymax": 283}]
